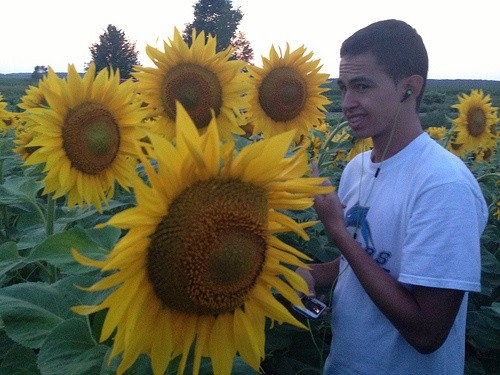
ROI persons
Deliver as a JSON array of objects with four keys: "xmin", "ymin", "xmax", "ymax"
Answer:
[{"xmin": 291, "ymin": 19, "xmax": 490, "ymax": 374}]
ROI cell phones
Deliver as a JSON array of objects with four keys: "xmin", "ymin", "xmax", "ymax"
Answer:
[{"xmin": 293, "ymin": 295, "xmax": 327, "ymax": 321}]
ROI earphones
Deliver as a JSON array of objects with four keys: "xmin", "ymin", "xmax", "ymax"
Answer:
[{"xmin": 400, "ymin": 89, "xmax": 412, "ymax": 104}]
